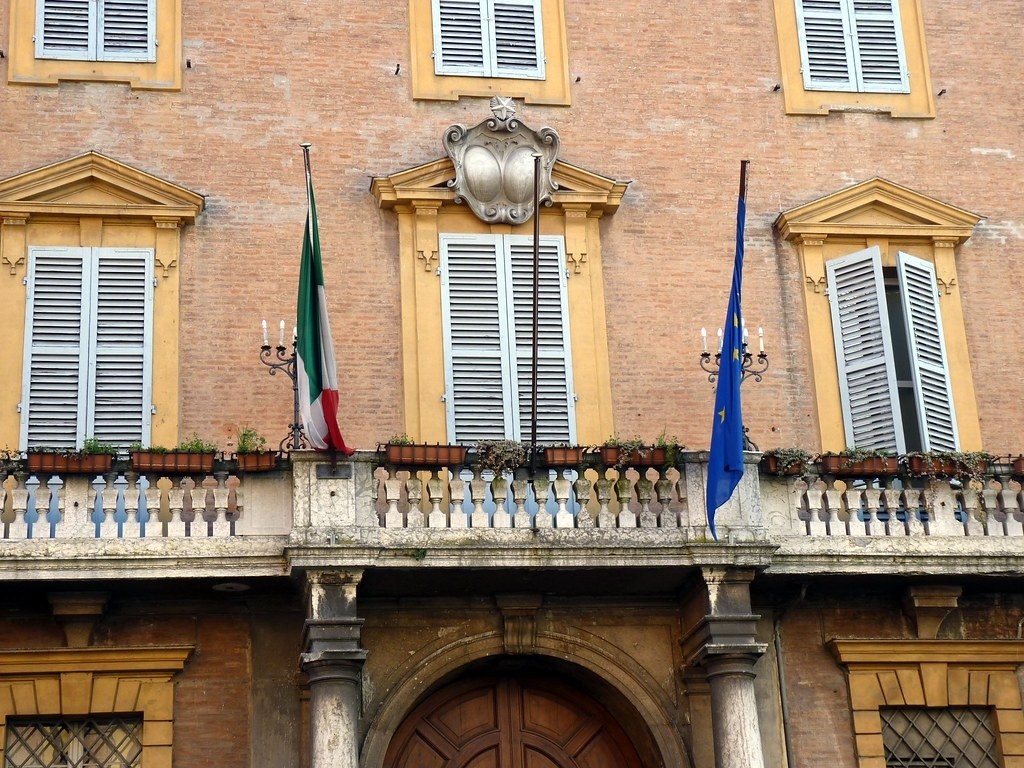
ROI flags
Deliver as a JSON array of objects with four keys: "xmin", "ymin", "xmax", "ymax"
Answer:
[
  {"xmin": 707, "ymin": 198, "xmax": 744, "ymax": 542},
  {"xmin": 297, "ymin": 171, "xmax": 357, "ymax": 454}
]
